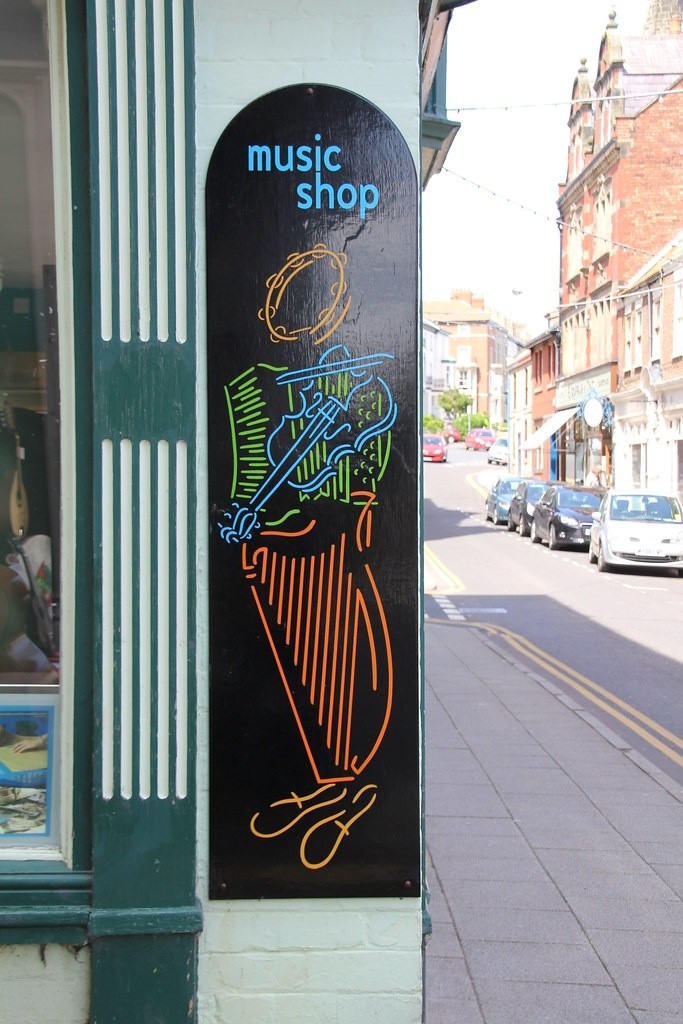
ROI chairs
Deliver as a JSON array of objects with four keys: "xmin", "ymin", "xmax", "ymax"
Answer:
[
  {"xmin": 649, "ymin": 502, "xmax": 658, "ymax": 511},
  {"xmin": 617, "ymin": 500, "xmax": 628, "ymax": 510}
]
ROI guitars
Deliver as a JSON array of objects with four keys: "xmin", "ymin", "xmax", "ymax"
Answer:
[
  {"xmin": 0, "ymin": 393, "xmax": 30, "ymax": 538},
  {"xmin": 11, "ymin": 540, "xmax": 55, "ymax": 653}
]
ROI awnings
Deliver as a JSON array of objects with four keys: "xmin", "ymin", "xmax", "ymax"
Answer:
[{"xmin": 518, "ymin": 408, "xmax": 581, "ymax": 454}]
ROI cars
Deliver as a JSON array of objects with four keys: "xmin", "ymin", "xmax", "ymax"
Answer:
[
  {"xmin": 530, "ymin": 485, "xmax": 617, "ymax": 550},
  {"xmin": 442, "ymin": 426, "xmax": 461, "ymax": 444},
  {"xmin": 487, "ymin": 437, "xmax": 508, "ymax": 465},
  {"xmin": 507, "ymin": 480, "xmax": 566, "ymax": 536},
  {"xmin": 465, "ymin": 429, "xmax": 496, "ymax": 452},
  {"xmin": 422, "ymin": 434, "xmax": 448, "ymax": 463},
  {"xmin": 485, "ymin": 478, "xmax": 526, "ymax": 525},
  {"xmin": 588, "ymin": 490, "xmax": 683, "ymax": 577}
]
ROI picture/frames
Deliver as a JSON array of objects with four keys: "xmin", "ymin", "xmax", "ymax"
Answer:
[{"xmin": 0, "ymin": 695, "xmax": 61, "ymax": 850}]
